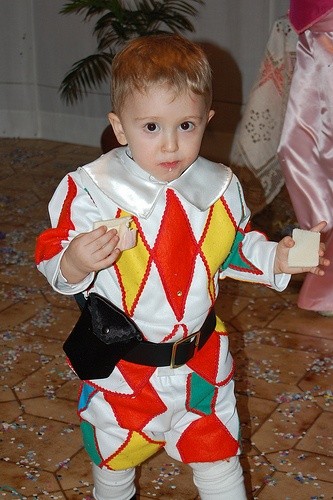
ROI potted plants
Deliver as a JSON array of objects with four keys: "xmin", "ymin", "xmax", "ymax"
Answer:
[{"xmin": 57, "ymin": 0, "xmax": 203, "ymax": 155}]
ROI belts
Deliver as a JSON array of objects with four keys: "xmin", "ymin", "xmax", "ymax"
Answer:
[{"xmin": 122, "ymin": 307, "xmax": 216, "ymax": 368}]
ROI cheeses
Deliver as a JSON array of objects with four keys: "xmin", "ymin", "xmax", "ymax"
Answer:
[
  {"xmin": 93, "ymin": 217, "xmax": 137, "ymax": 252},
  {"xmin": 287, "ymin": 228, "xmax": 320, "ymax": 266}
]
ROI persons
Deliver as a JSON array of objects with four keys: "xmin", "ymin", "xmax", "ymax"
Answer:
[
  {"xmin": 35, "ymin": 33, "xmax": 331, "ymax": 500},
  {"xmin": 276, "ymin": 0, "xmax": 333, "ymax": 316}
]
